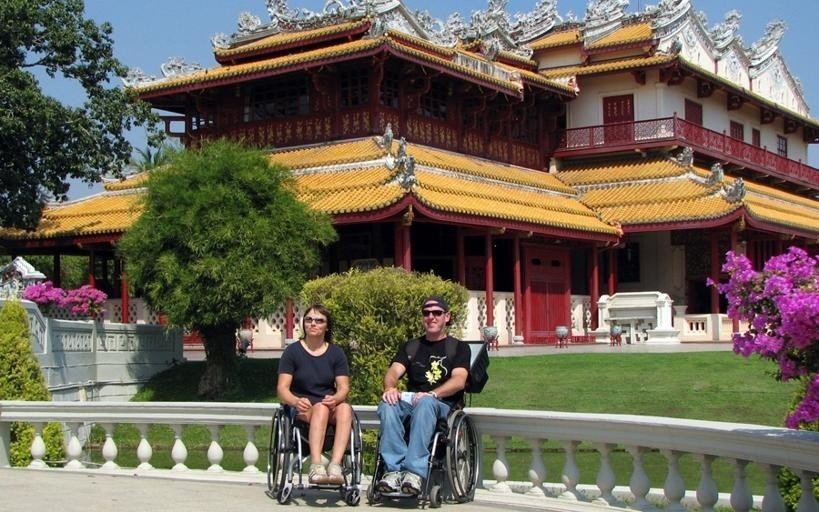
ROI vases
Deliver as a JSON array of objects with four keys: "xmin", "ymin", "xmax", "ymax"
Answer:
[
  {"xmin": 239, "ymin": 330, "xmax": 254, "ymax": 343},
  {"xmin": 482, "ymin": 326, "xmax": 622, "ymax": 341}
]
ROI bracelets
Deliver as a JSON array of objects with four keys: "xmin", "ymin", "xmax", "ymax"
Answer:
[{"xmin": 428, "ymin": 390, "xmax": 438, "ymax": 399}]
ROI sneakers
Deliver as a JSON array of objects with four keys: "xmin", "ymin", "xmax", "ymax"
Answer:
[
  {"xmin": 375, "ymin": 470, "xmax": 423, "ymax": 494},
  {"xmin": 309, "ymin": 463, "xmax": 346, "ymax": 484}
]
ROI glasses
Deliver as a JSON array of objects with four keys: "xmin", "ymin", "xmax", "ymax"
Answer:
[
  {"xmin": 303, "ymin": 316, "xmax": 325, "ymax": 324},
  {"xmin": 422, "ymin": 310, "xmax": 447, "ymax": 317}
]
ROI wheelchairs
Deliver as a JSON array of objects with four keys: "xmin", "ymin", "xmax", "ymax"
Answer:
[
  {"xmin": 265, "ymin": 339, "xmax": 363, "ymax": 506},
  {"xmin": 367, "ymin": 341, "xmax": 490, "ymax": 507}
]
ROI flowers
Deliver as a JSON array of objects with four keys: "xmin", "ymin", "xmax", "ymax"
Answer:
[
  {"xmin": 22, "ymin": 280, "xmax": 108, "ymax": 314},
  {"xmin": 706, "ymin": 246, "xmax": 819, "ymax": 431}
]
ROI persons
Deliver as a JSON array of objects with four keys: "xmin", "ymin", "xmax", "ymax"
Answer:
[
  {"xmin": 378, "ymin": 297, "xmax": 471, "ymax": 495},
  {"xmin": 276, "ymin": 303, "xmax": 352, "ymax": 485}
]
